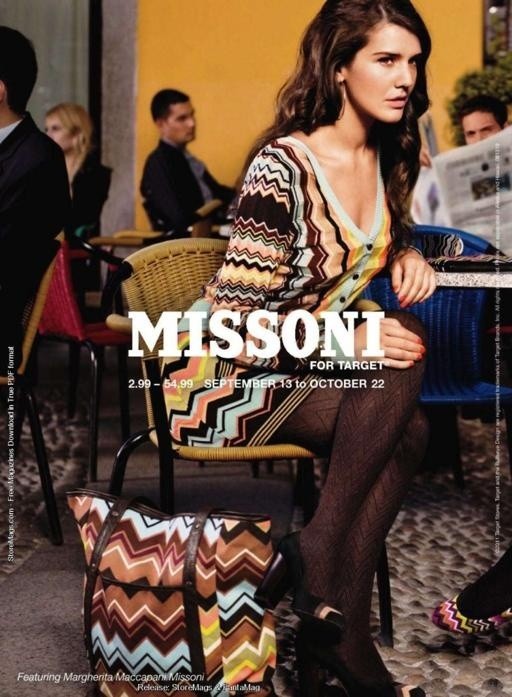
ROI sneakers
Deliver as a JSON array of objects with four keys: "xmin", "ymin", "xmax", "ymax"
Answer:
[{"xmin": 432, "ymin": 591, "xmax": 512, "ymax": 634}]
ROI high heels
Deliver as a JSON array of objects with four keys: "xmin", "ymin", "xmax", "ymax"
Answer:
[
  {"xmin": 254, "ymin": 530, "xmax": 344, "ymax": 635},
  {"xmin": 294, "ymin": 623, "xmax": 425, "ymax": 696}
]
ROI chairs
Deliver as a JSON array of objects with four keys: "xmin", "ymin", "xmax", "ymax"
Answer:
[{"xmin": 2, "ymin": 199, "xmax": 511, "ymax": 649}]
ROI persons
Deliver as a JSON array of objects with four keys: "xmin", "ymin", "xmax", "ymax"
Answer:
[
  {"xmin": 433, "ymin": 542, "xmax": 512, "ymax": 639},
  {"xmin": 417, "ymin": 96, "xmax": 510, "ymax": 167},
  {"xmin": 138, "ymin": 87, "xmax": 236, "ymax": 236},
  {"xmin": 159, "ymin": 1, "xmax": 443, "ymax": 697},
  {"xmin": 42, "ymin": 103, "xmax": 113, "ymax": 318},
  {"xmin": 0, "ymin": 26, "xmax": 70, "ymax": 377}
]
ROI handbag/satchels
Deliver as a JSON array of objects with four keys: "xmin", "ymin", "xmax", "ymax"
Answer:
[{"xmin": 65, "ymin": 488, "xmax": 278, "ymax": 697}]
